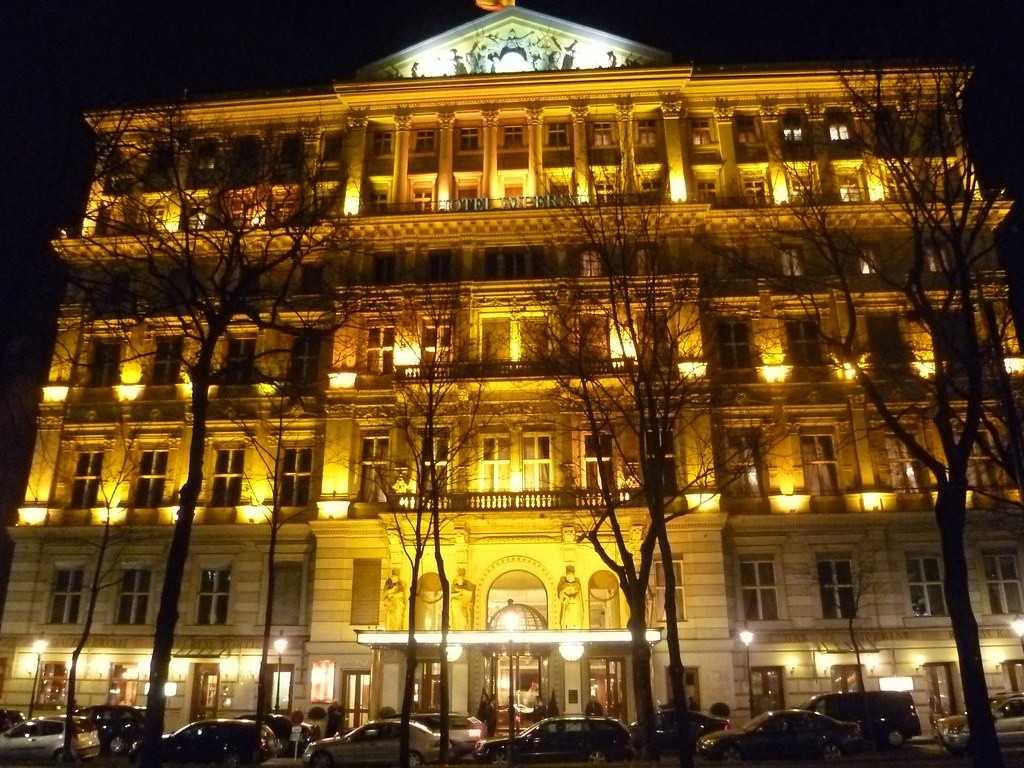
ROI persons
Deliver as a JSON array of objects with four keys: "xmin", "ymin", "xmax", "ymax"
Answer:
[
  {"xmin": 928, "ymin": 695, "xmax": 942, "ymax": 738},
  {"xmin": 585, "ymin": 695, "xmax": 604, "ymax": 716},
  {"xmin": 486, "ymin": 699, "xmax": 497, "ymax": 739},
  {"xmin": 687, "ymin": 695, "xmax": 699, "ymax": 712},
  {"xmin": 477, "ymin": 693, "xmax": 489, "ymax": 724},
  {"xmin": 324, "ymin": 700, "xmax": 344, "ymax": 739},
  {"xmin": 532, "ymin": 696, "xmax": 546, "ymax": 724}
]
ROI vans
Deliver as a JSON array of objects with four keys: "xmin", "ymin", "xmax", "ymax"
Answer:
[{"xmin": 798, "ymin": 688, "xmax": 922, "ymax": 749}]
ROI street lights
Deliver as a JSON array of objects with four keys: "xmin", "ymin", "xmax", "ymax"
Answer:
[
  {"xmin": 1012, "ymin": 613, "xmax": 1024, "ymax": 654},
  {"xmin": 273, "ymin": 629, "xmax": 288, "ymax": 714},
  {"xmin": 738, "ymin": 621, "xmax": 755, "ymax": 720},
  {"xmin": 27, "ymin": 633, "xmax": 49, "ymax": 720}
]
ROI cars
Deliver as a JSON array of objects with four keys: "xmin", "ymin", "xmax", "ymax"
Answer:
[
  {"xmin": 471, "ymin": 715, "xmax": 635, "ymax": 767},
  {"xmin": 697, "ymin": 708, "xmax": 866, "ymax": 765},
  {"xmin": 384, "ymin": 710, "xmax": 487, "ymax": 754},
  {"xmin": 232, "ymin": 713, "xmax": 320, "ymax": 756},
  {"xmin": 301, "ymin": 717, "xmax": 453, "ymax": 768},
  {"xmin": 62, "ymin": 704, "xmax": 147, "ymax": 754},
  {"xmin": 0, "ymin": 709, "xmax": 26, "ymax": 732},
  {"xmin": 938, "ymin": 691, "xmax": 1024, "ymax": 753},
  {"xmin": 630, "ymin": 709, "xmax": 731, "ymax": 754},
  {"xmin": 127, "ymin": 717, "xmax": 278, "ymax": 768},
  {"xmin": 0, "ymin": 714, "xmax": 101, "ymax": 764}
]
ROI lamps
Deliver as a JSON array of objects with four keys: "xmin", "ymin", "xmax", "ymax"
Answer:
[
  {"xmin": 434, "ymin": 644, "xmax": 463, "ymax": 662},
  {"xmin": 558, "ymin": 642, "xmax": 584, "ymax": 661}
]
